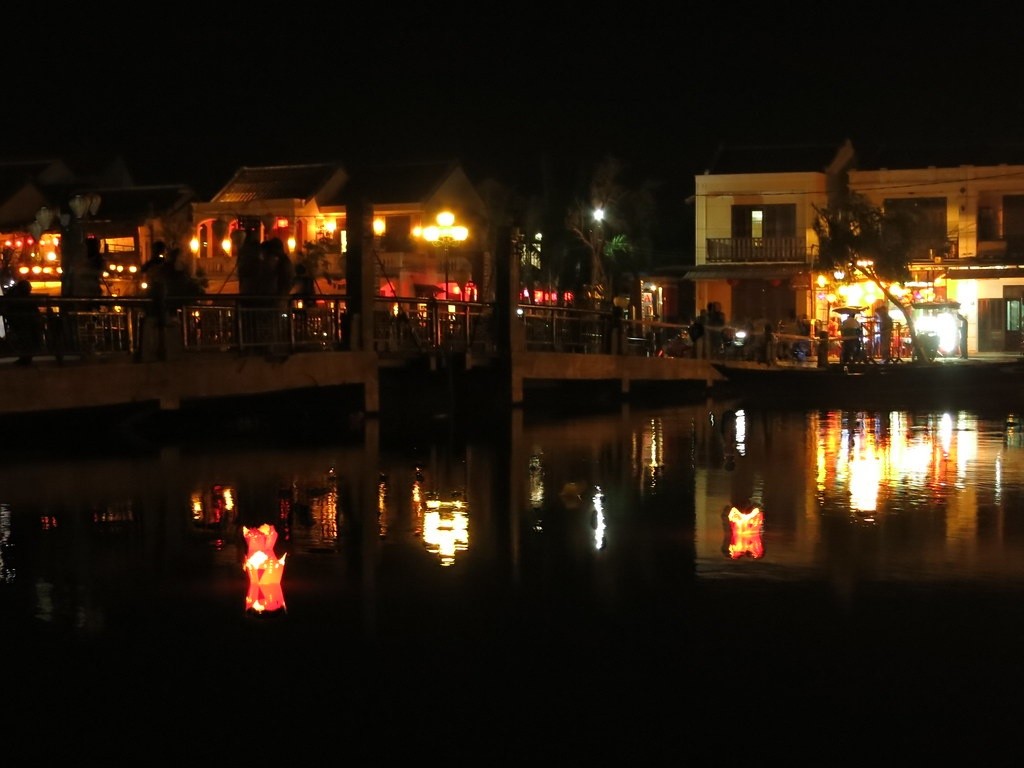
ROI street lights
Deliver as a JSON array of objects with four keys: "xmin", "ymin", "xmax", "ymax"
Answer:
[
  {"xmin": 28, "ymin": 190, "xmax": 100, "ymax": 305},
  {"xmin": 426, "ymin": 210, "xmax": 469, "ymax": 318}
]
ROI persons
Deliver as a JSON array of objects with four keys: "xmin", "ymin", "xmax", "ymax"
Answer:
[
  {"xmin": 696, "ymin": 301, "xmax": 725, "ymax": 357},
  {"xmin": 261, "ymin": 236, "xmax": 316, "ymax": 340},
  {"xmin": 0, "ymin": 238, "xmax": 108, "ymax": 354},
  {"xmin": 959, "ymin": 315, "xmax": 968, "ymax": 359},
  {"xmin": 765, "ymin": 319, "xmax": 782, "ymax": 360},
  {"xmin": 141, "ymin": 241, "xmax": 184, "ymax": 315},
  {"xmin": 843, "ymin": 312, "xmax": 862, "ymax": 354},
  {"xmin": 873, "ymin": 307, "xmax": 893, "ymax": 364}
]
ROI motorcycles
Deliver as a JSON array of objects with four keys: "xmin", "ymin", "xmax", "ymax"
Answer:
[{"xmin": 911, "ymin": 329, "xmax": 940, "ymax": 364}]
